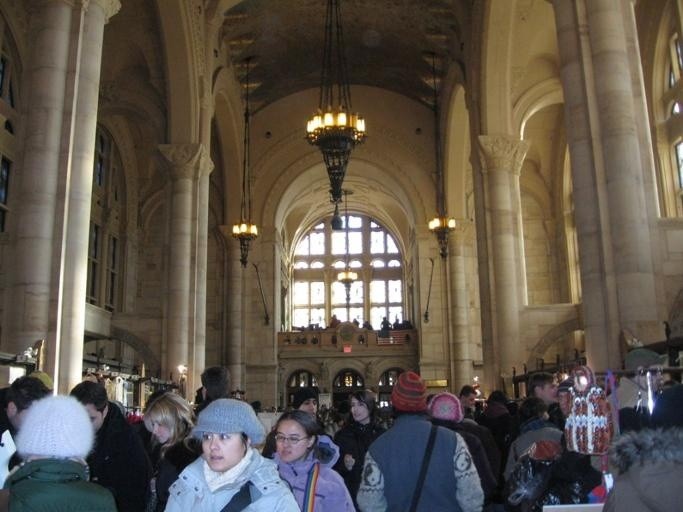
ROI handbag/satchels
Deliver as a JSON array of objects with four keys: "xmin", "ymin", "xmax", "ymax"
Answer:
[
  {"xmin": 619, "ymin": 407, "xmax": 649, "ymax": 434},
  {"xmin": 565, "ymin": 365, "xmax": 616, "ymax": 455}
]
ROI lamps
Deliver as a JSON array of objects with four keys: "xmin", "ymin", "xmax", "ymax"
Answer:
[
  {"xmin": 232, "ymin": 57, "xmax": 257, "ymax": 268},
  {"xmin": 306, "ymin": 0, "xmax": 367, "ymax": 230},
  {"xmin": 427, "ymin": 53, "xmax": 457, "ymax": 260},
  {"xmin": 336, "ymin": 189, "xmax": 359, "ymax": 303}
]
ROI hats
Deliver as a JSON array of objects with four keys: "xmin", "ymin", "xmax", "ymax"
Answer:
[
  {"xmin": 624, "ymin": 349, "xmax": 667, "ymax": 370},
  {"xmin": 193, "ymin": 399, "xmax": 266, "ymax": 445},
  {"xmin": 293, "ymin": 387, "xmax": 317, "ymax": 409},
  {"xmin": 29, "ymin": 372, "xmax": 54, "ymax": 390},
  {"xmin": 16, "ymin": 396, "xmax": 94, "ymax": 457},
  {"xmin": 392, "ymin": 371, "xmax": 426, "ymax": 412}
]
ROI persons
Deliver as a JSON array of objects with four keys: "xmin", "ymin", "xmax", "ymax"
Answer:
[
  {"xmin": 604, "ymin": 383, "xmax": 683, "ymax": 512},
  {"xmin": 1, "ymin": 365, "xmax": 603, "ymax": 512},
  {"xmin": 296, "ymin": 314, "xmax": 414, "ymax": 344}
]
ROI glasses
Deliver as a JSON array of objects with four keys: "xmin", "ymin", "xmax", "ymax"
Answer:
[{"xmin": 274, "ymin": 436, "xmax": 310, "ymax": 444}]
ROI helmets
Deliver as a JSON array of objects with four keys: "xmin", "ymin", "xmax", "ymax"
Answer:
[{"xmin": 426, "ymin": 392, "xmax": 465, "ymax": 423}]
